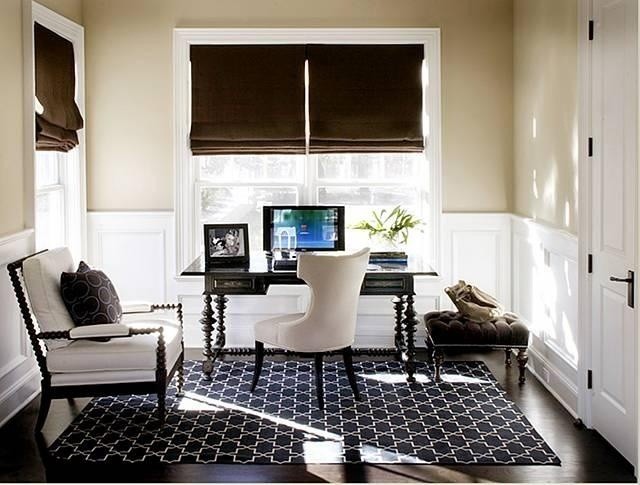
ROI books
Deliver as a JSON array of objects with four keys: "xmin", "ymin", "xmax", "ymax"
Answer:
[{"xmin": 368, "ymin": 251, "xmax": 408, "ymax": 267}]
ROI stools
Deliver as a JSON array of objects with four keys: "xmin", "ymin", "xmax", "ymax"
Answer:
[{"xmin": 423, "ymin": 312, "xmax": 530, "ymax": 391}]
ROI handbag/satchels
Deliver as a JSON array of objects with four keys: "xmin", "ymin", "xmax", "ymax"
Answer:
[{"xmin": 443, "ymin": 280, "xmax": 504, "ymax": 323}]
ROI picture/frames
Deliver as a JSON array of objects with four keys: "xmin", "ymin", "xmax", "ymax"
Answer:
[{"xmin": 204, "ymin": 222, "xmax": 250, "ymax": 271}]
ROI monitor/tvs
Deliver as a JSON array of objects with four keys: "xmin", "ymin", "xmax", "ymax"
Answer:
[{"xmin": 263, "ymin": 205, "xmax": 346, "ymax": 252}]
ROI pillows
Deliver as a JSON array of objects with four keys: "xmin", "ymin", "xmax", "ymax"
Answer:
[{"xmin": 58, "ymin": 258, "xmax": 123, "ymax": 343}]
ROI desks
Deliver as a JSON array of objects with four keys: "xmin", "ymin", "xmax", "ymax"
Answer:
[{"xmin": 181, "ymin": 243, "xmax": 438, "ymax": 386}]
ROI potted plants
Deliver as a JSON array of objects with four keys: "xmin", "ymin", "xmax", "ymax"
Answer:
[{"xmin": 346, "ymin": 203, "xmax": 424, "ymax": 251}]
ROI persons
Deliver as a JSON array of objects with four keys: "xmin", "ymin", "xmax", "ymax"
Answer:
[{"xmin": 210, "ymin": 228, "xmax": 240, "ymax": 255}]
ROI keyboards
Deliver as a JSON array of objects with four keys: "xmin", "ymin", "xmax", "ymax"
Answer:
[{"xmin": 273, "ymin": 260, "xmax": 298, "ymax": 271}]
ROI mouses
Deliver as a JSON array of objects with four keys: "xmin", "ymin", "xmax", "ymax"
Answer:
[{"xmin": 368, "ymin": 264, "xmax": 378, "ymax": 272}]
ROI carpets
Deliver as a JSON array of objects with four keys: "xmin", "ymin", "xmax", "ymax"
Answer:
[{"xmin": 42, "ymin": 359, "xmax": 563, "ymax": 468}]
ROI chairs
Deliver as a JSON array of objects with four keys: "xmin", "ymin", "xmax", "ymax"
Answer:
[
  {"xmin": 8, "ymin": 246, "xmax": 186, "ymax": 433},
  {"xmin": 250, "ymin": 246, "xmax": 373, "ymax": 412}
]
